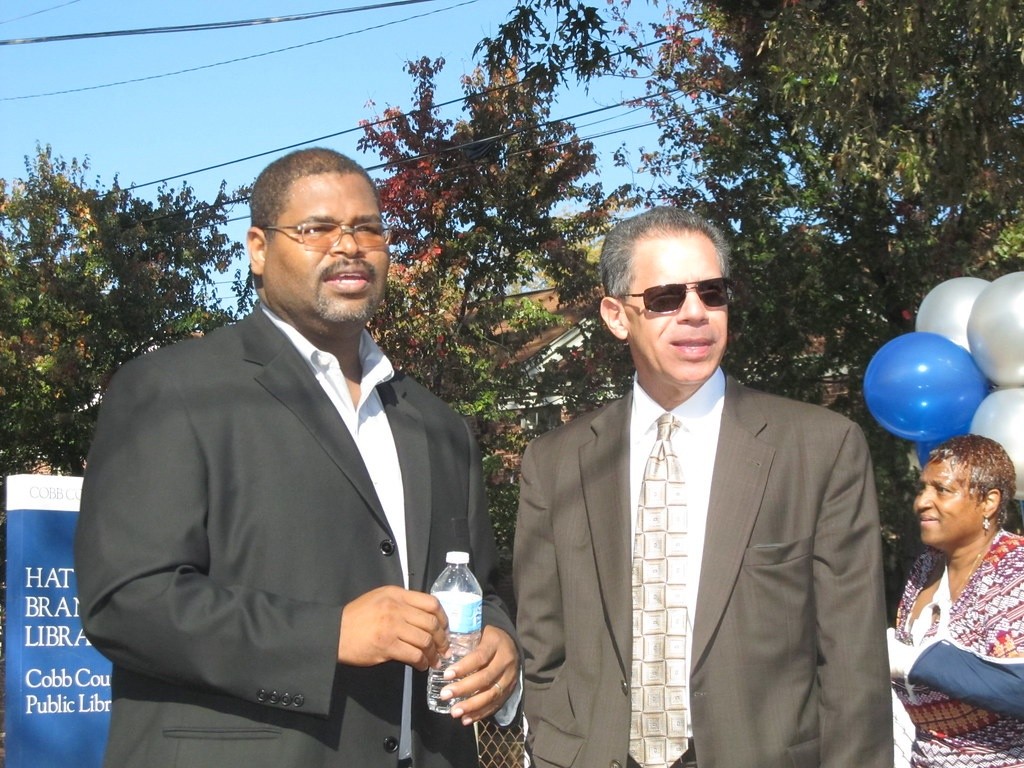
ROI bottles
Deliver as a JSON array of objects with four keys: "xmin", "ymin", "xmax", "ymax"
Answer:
[{"xmin": 427, "ymin": 550, "xmax": 483, "ymax": 714}]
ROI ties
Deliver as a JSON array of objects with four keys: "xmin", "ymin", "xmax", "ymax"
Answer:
[{"xmin": 629, "ymin": 415, "xmax": 689, "ymax": 768}]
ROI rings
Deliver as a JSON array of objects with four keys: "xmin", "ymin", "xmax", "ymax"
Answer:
[{"xmin": 493, "ymin": 682, "xmax": 502, "ymax": 692}]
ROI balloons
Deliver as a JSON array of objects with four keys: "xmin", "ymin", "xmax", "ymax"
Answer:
[{"xmin": 863, "ymin": 269, "xmax": 1024, "ymax": 501}]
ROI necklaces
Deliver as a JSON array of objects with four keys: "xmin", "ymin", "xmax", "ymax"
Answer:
[{"xmin": 952, "ymin": 531, "xmax": 997, "ymax": 598}]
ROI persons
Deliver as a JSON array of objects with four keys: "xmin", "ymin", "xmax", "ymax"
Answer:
[
  {"xmin": 513, "ymin": 207, "xmax": 896, "ymax": 767},
  {"xmin": 74, "ymin": 146, "xmax": 525, "ymax": 768},
  {"xmin": 887, "ymin": 435, "xmax": 1024, "ymax": 768}
]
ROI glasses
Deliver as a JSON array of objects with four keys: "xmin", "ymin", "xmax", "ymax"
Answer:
[
  {"xmin": 263, "ymin": 222, "xmax": 396, "ymax": 247},
  {"xmin": 615, "ymin": 277, "xmax": 734, "ymax": 314}
]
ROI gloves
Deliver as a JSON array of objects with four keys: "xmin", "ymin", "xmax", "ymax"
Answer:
[{"xmin": 886, "ymin": 628, "xmax": 927, "ymax": 680}]
